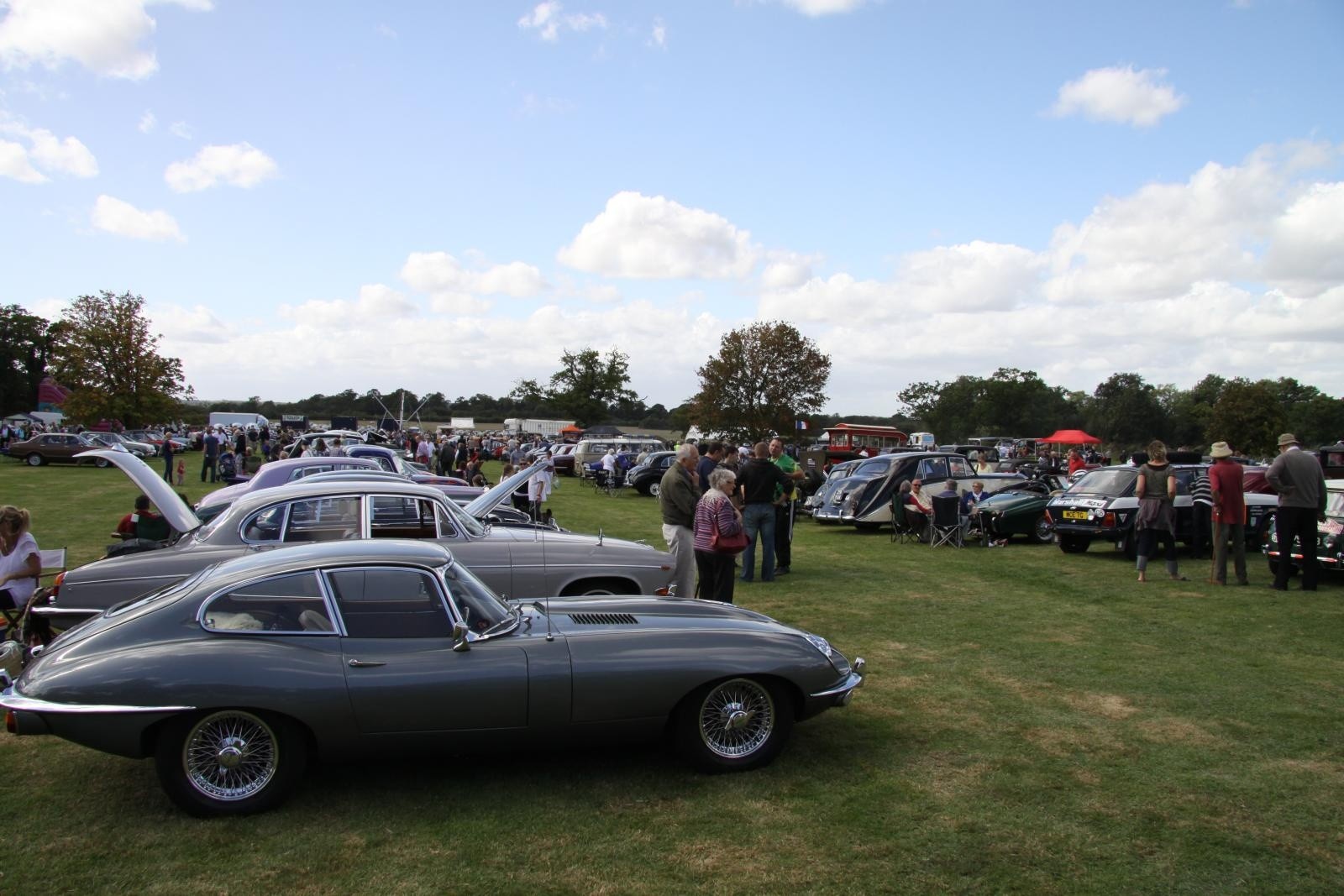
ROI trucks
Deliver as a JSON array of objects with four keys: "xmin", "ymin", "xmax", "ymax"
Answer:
[{"xmin": 210, "ymin": 413, "xmax": 269, "ymax": 427}]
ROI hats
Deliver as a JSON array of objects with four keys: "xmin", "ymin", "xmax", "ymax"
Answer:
[
  {"xmin": 1210, "ymin": 442, "xmax": 1233, "ymax": 458},
  {"xmin": 1277, "ymin": 433, "xmax": 1300, "ymax": 445}
]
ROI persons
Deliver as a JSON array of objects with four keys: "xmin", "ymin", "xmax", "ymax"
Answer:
[
  {"xmin": 599, "ymin": 437, "xmax": 749, "ymax": 569},
  {"xmin": 117, "ymin": 493, "xmax": 196, "ymax": 533},
  {"xmin": 660, "ymin": 443, "xmax": 699, "ymax": 598},
  {"xmin": 768, "ymin": 438, "xmax": 803, "ymax": 576},
  {"xmin": 0, "ymin": 504, "xmax": 64, "ymax": 640},
  {"xmin": 0, "ymin": 421, "xmax": 86, "ymax": 457},
  {"xmin": 1265, "ymin": 434, "xmax": 1328, "ymax": 591},
  {"xmin": 1206, "ymin": 441, "xmax": 1250, "ymax": 586},
  {"xmin": 162, "ymin": 431, "xmax": 175, "ymax": 486},
  {"xmin": 693, "ymin": 469, "xmax": 742, "ymax": 602},
  {"xmin": 1235, "ymin": 450, "xmax": 1248, "ymax": 459},
  {"xmin": 176, "ymin": 459, "xmax": 186, "ymax": 486},
  {"xmin": 1135, "ymin": 440, "xmax": 1183, "ymax": 580},
  {"xmin": 196, "ymin": 422, "xmax": 557, "ymax": 522},
  {"xmin": 730, "ymin": 442, "xmax": 795, "ymax": 582},
  {"xmin": 146, "ymin": 416, "xmax": 188, "ymax": 435},
  {"xmin": 821, "ymin": 433, "xmax": 1129, "ymax": 585}
]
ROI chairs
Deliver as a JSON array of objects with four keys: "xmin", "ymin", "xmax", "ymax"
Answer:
[
  {"xmin": 889, "ymin": 491, "xmax": 927, "ymax": 544},
  {"xmin": 961, "ymin": 487, "xmax": 994, "ymax": 546},
  {"xmin": 349, "ymin": 532, "xmax": 362, "ymax": 539},
  {"xmin": 0, "ymin": 546, "xmax": 69, "ymax": 641},
  {"xmin": 298, "ymin": 609, "xmax": 335, "ymax": 633},
  {"xmin": 929, "ymin": 494, "xmax": 964, "ymax": 551},
  {"xmin": 580, "ymin": 461, "xmax": 597, "ymax": 488},
  {"xmin": 109, "ymin": 514, "xmax": 170, "ymax": 542},
  {"xmin": 339, "ymin": 527, "xmax": 358, "ymax": 538},
  {"xmin": 604, "ymin": 476, "xmax": 624, "ymax": 497},
  {"xmin": 595, "ymin": 469, "xmax": 610, "ymax": 496}
]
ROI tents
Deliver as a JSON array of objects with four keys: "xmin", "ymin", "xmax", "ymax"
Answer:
[
  {"xmin": 560, "ymin": 424, "xmax": 584, "ymax": 436},
  {"xmin": 1035, "ymin": 430, "xmax": 1103, "ymax": 461}
]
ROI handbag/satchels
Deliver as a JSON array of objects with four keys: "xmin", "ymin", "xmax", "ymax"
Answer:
[{"xmin": 710, "ymin": 498, "xmax": 751, "ymax": 555}]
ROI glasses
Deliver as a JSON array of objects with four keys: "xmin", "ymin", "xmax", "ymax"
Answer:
[
  {"xmin": 913, "ymin": 484, "xmax": 922, "ymax": 488},
  {"xmin": 972, "ymin": 485, "xmax": 978, "ymax": 488}
]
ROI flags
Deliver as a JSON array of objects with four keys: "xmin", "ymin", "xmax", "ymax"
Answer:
[{"xmin": 795, "ymin": 420, "xmax": 809, "ymax": 430}]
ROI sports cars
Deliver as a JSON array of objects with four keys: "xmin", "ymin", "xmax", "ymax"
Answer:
[{"xmin": 0, "ymin": 538, "xmax": 866, "ymax": 817}]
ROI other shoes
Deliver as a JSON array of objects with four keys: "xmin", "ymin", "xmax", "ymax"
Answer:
[
  {"xmin": 1169, "ymin": 576, "xmax": 1191, "ymax": 581},
  {"xmin": 773, "ymin": 565, "xmax": 790, "ymax": 575},
  {"xmin": 1291, "ymin": 585, "xmax": 1317, "ymax": 591},
  {"xmin": 1264, "ymin": 583, "xmax": 1288, "ymax": 591},
  {"xmin": 1238, "ymin": 580, "xmax": 1249, "ymax": 586},
  {"xmin": 1209, "ymin": 578, "xmax": 1226, "ymax": 585},
  {"xmin": 1136, "ymin": 577, "xmax": 1145, "ymax": 581}
]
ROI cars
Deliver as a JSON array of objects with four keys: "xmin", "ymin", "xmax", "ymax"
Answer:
[
  {"xmin": 31, "ymin": 443, "xmax": 676, "ymax": 599},
  {"xmin": 0, "ymin": 429, "xmax": 192, "ymax": 469},
  {"xmin": 193, "ymin": 428, "xmax": 532, "ymax": 527},
  {"xmin": 526, "ymin": 435, "xmax": 677, "ymax": 497},
  {"xmin": 795, "ymin": 423, "xmax": 1344, "ymax": 585}
]
